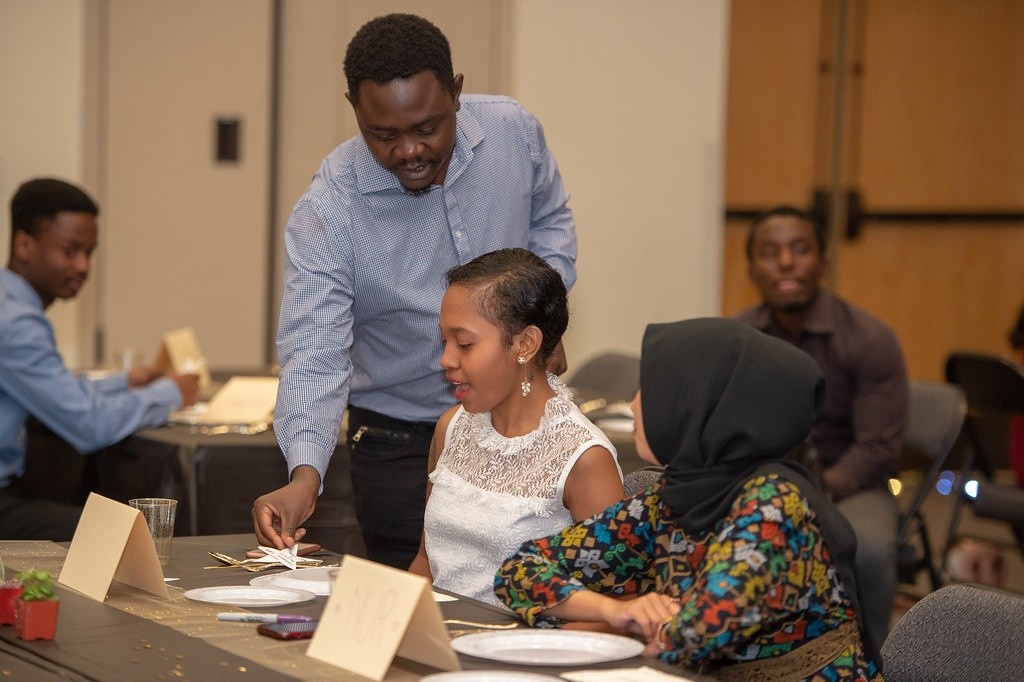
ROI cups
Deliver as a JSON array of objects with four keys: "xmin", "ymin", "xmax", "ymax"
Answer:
[{"xmin": 128, "ymin": 498, "xmax": 179, "ymax": 566}]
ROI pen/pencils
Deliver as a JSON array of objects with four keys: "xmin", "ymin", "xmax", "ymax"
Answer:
[{"xmin": 216, "ymin": 612, "xmax": 314, "ymax": 623}]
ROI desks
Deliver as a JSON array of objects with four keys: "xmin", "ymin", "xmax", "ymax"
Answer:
[
  {"xmin": 0, "ymin": 532, "xmax": 717, "ymax": 682},
  {"xmin": 88, "ymin": 368, "xmax": 346, "ymax": 536}
]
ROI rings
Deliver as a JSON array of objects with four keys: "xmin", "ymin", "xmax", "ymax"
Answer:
[{"xmin": 666, "ymin": 597, "xmax": 680, "ymax": 610}]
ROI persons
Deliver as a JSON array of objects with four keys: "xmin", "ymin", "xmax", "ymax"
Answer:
[
  {"xmin": 252, "ymin": 13, "xmax": 579, "ymax": 572},
  {"xmin": 0, "ymin": 178, "xmax": 201, "ymax": 541},
  {"xmin": 404, "ymin": 206, "xmax": 915, "ymax": 682}
]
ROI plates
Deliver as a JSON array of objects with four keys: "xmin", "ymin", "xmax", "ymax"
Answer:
[
  {"xmin": 448, "ymin": 629, "xmax": 647, "ymax": 666},
  {"xmin": 249, "ymin": 566, "xmax": 342, "ymax": 596},
  {"xmin": 418, "ymin": 670, "xmax": 573, "ymax": 682},
  {"xmin": 184, "ymin": 585, "xmax": 317, "ymax": 608}
]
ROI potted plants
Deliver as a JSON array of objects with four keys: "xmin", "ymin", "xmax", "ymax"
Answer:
[
  {"xmin": 0, "ymin": 557, "xmax": 24, "ymax": 626},
  {"xmin": 14, "ymin": 567, "xmax": 61, "ymax": 641}
]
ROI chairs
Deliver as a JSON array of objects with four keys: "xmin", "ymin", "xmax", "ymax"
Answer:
[{"xmin": 566, "ymin": 348, "xmax": 1024, "ymax": 682}]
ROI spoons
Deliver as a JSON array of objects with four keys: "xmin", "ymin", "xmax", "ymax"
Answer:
[
  {"xmin": 208, "ymin": 550, "xmax": 324, "ymax": 565},
  {"xmin": 228, "ymin": 559, "xmax": 339, "ymax": 572}
]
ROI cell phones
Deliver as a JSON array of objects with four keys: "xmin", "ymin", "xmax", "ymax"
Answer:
[{"xmin": 257, "ymin": 621, "xmax": 319, "ymax": 641}]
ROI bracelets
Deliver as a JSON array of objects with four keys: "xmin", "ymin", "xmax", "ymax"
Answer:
[{"xmin": 653, "ymin": 616, "xmax": 673, "ymax": 652}]
